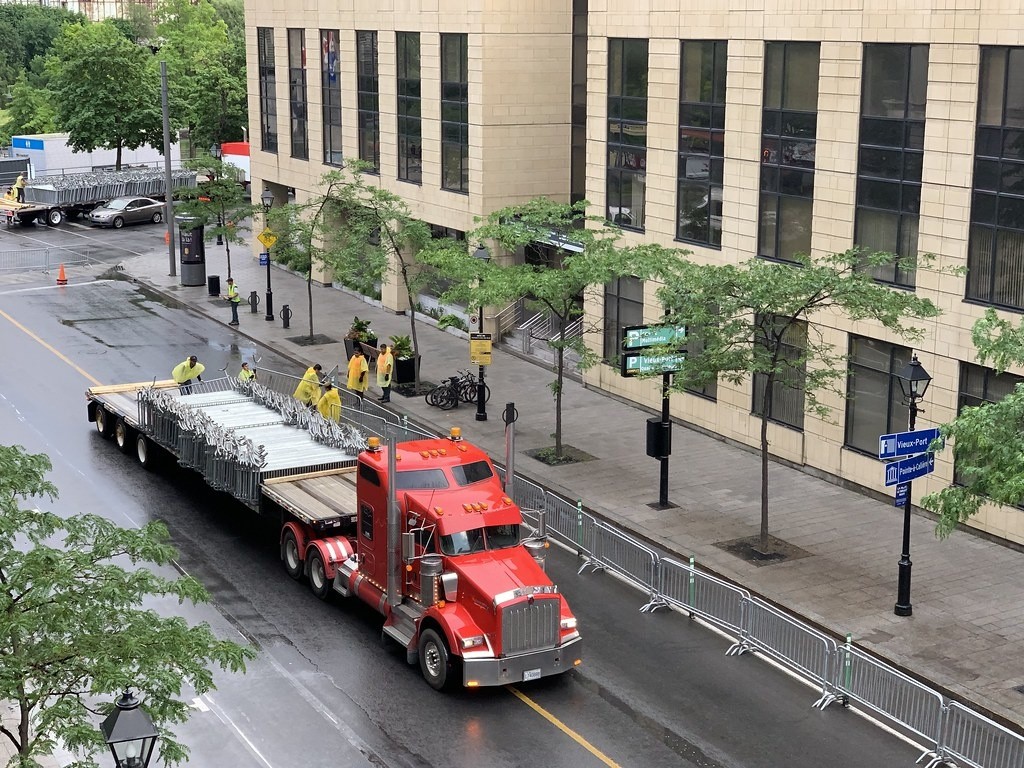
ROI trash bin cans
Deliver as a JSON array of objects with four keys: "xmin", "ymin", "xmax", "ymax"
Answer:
[{"xmin": 208, "ymin": 275, "xmax": 221, "ymax": 296}]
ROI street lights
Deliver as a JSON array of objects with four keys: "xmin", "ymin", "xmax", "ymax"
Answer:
[
  {"xmin": 209, "ymin": 141, "xmax": 224, "ymax": 245},
  {"xmin": 241, "ymin": 126, "xmax": 248, "ymax": 142},
  {"xmin": 260, "ymin": 185, "xmax": 277, "ymax": 322},
  {"xmin": 888, "ymin": 354, "xmax": 934, "ymax": 618}
]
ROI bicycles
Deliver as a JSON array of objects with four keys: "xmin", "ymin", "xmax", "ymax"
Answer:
[{"xmin": 425, "ymin": 368, "xmax": 491, "ymax": 410}]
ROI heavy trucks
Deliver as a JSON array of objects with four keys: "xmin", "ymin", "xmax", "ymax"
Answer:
[
  {"xmin": 0, "ymin": 166, "xmax": 197, "ymax": 228},
  {"xmin": 86, "ymin": 358, "xmax": 585, "ymax": 694}
]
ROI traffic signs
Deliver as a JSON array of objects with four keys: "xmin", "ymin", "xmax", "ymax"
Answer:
[
  {"xmin": 885, "ymin": 450, "xmax": 935, "ymax": 486},
  {"xmin": 878, "ymin": 426, "xmax": 945, "ymax": 459},
  {"xmin": 621, "ymin": 320, "xmax": 690, "ymax": 350},
  {"xmin": 621, "ymin": 349, "xmax": 688, "ymax": 378}
]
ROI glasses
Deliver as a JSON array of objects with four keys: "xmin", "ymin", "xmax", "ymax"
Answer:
[{"xmin": 193, "ymin": 361, "xmax": 197, "ymax": 362}]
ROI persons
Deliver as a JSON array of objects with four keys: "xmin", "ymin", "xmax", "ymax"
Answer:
[
  {"xmin": 238, "ymin": 362, "xmax": 255, "ymax": 382},
  {"xmin": 293, "ymin": 364, "xmax": 322, "ymax": 413},
  {"xmin": 172, "ymin": 356, "xmax": 205, "ymax": 396},
  {"xmin": 317, "ymin": 382, "xmax": 341, "ymax": 424},
  {"xmin": 375, "ymin": 344, "xmax": 393, "ymax": 403},
  {"xmin": 4, "ymin": 191, "xmax": 18, "ymax": 225},
  {"xmin": 17, "ymin": 172, "xmax": 26, "ymax": 203},
  {"xmin": 346, "ymin": 347, "xmax": 369, "ymax": 406},
  {"xmin": 225, "ymin": 278, "xmax": 240, "ymax": 325}
]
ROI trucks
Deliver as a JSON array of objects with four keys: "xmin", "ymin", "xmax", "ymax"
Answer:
[
  {"xmin": 221, "ymin": 142, "xmax": 251, "ymax": 202},
  {"xmin": 11, "ymin": 129, "xmax": 181, "ymax": 180},
  {"xmin": 0, "ymin": 152, "xmax": 35, "ymax": 194}
]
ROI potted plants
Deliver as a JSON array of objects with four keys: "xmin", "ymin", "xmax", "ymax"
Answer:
[
  {"xmin": 389, "ymin": 334, "xmax": 421, "ymax": 384},
  {"xmin": 344, "ymin": 315, "xmax": 378, "ymax": 361}
]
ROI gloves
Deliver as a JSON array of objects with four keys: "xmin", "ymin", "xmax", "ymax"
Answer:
[
  {"xmin": 359, "ymin": 377, "xmax": 363, "ymax": 382},
  {"xmin": 227, "ymin": 297, "xmax": 232, "ymax": 301},
  {"xmin": 253, "ymin": 369, "xmax": 256, "ymax": 373},
  {"xmin": 320, "ymin": 380, "xmax": 325, "ymax": 386},
  {"xmin": 385, "ymin": 373, "xmax": 389, "ymax": 381}
]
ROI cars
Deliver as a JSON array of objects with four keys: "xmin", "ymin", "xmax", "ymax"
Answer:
[
  {"xmin": 175, "ymin": 210, "xmax": 209, "ymax": 225},
  {"xmin": 88, "ymin": 196, "xmax": 166, "ymax": 229}
]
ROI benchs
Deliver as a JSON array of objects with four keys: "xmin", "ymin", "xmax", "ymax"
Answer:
[{"xmin": 359, "ymin": 341, "xmax": 381, "ymax": 373}]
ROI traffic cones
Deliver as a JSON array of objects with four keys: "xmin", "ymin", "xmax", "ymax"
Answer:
[
  {"xmin": 56, "ymin": 263, "xmax": 67, "ymax": 285},
  {"xmin": 165, "ymin": 231, "xmax": 170, "ymax": 244}
]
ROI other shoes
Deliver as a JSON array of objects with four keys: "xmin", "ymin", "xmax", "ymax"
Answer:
[
  {"xmin": 229, "ymin": 320, "xmax": 239, "ymax": 325},
  {"xmin": 382, "ymin": 399, "xmax": 390, "ymax": 403},
  {"xmin": 378, "ymin": 397, "xmax": 384, "ymax": 400}
]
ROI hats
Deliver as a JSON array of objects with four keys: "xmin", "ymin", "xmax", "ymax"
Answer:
[
  {"xmin": 190, "ymin": 356, "xmax": 197, "ymax": 361},
  {"xmin": 226, "ymin": 278, "xmax": 233, "ymax": 282},
  {"xmin": 324, "ymin": 382, "xmax": 331, "ymax": 387}
]
